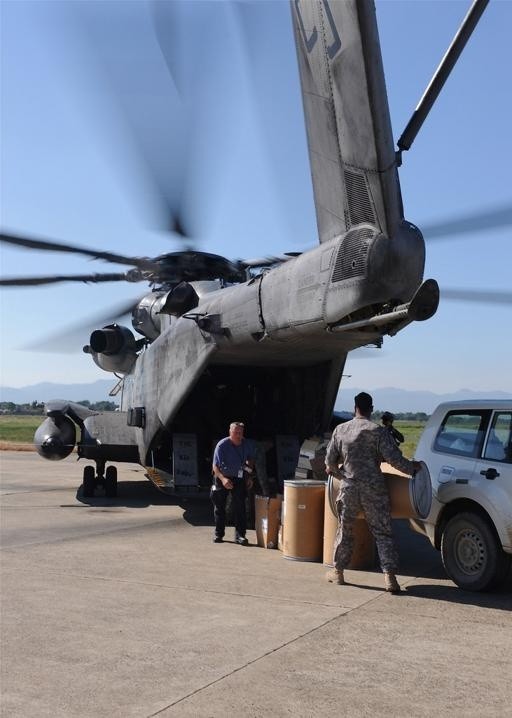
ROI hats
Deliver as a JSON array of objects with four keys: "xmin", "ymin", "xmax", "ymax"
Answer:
[{"xmin": 380, "ymin": 412, "xmax": 393, "ymax": 419}]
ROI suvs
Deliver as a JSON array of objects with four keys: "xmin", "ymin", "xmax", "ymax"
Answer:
[{"xmin": 408, "ymin": 398, "xmax": 512, "ymax": 594}]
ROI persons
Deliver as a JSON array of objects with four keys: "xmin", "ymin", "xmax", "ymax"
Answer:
[
  {"xmin": 324, "ymin": 391, "xmax": 423, "ymax": 592},
  {"xmin": 379, "ymin": 411, "xmax": 405, "ymax": 461},
  {"xmin": 210, "ymin": 401, "xmax": 270, "ymax": 546}
]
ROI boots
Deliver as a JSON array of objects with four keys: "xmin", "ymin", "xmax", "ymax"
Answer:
[
  {"xmin": 385, "ymin": 573, "xmax": 400, "ymax": 592},
  {"xmin": 327, "ymin": 567, "xmax": 344, "ymax": 585}
]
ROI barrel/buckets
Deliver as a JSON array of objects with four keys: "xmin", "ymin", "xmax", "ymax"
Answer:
[
  {"xmin": 281, "ymin": 477, "xmax": 326, "ymax": 562},
  {"xmin": 329, "ymin": 460, "xmax": 432, "ymax": 521},
  {"xmin": 322, "ymin": 482, "xmax": 376, "ymax": 570}
]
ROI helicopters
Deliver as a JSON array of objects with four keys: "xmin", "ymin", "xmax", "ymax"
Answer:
[{"xmin": 1, "ymin": 2, "xmax": 488, "ymax": 499}]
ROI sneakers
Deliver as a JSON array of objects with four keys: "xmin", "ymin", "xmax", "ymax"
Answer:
[
  {"xmin": 213, "ymin": 536, "xmax": 222, "ymax": 542},
  {"xmin": 235, "ymin": 537, "xmax": 248, "ymax": 545}
]
